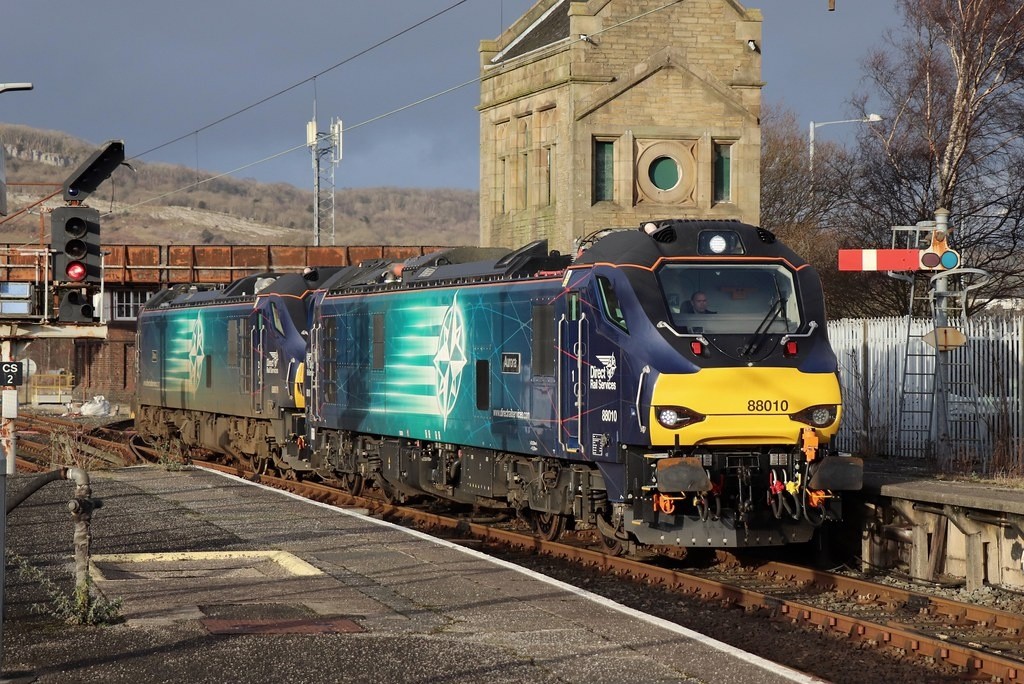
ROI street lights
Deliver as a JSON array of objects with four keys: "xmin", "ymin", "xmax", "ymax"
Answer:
[{"xmin": 808, "ymin": 112, "xmax": 884, "ymax": 217}]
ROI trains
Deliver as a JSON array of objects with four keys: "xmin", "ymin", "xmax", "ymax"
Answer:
[{"xmin": 132, "ymin": 218, "xmax": 864, "ymax": 563}]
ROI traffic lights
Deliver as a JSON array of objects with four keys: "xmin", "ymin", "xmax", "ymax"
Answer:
[
  {"xmin": 52, "ymin": 207, "xmax": 100, "ymax": 282},
  {"xmin": 62, "ymin": 139, "xmax": 125, "ymax": 200}
]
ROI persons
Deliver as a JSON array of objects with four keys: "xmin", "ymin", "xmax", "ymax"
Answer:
[{"xmin": 687, "ymin": 291, "xmax": 717, "ymax": 314}]
